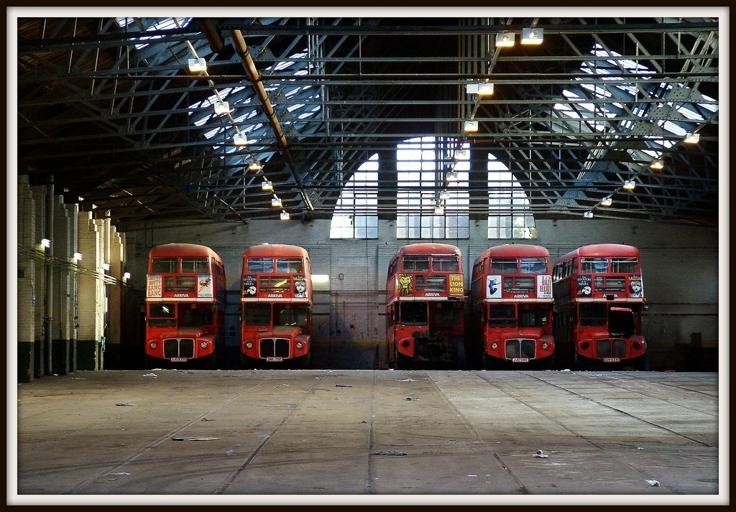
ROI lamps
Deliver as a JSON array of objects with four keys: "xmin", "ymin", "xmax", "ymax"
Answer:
[
  {"xmin": 600, "ymin": 132, "xmax": 700, "ymax": 207},
  {"xmin": 432, "ymin": 27, "xmax": 543, "ymax": 216},
  {"xmin": 183, "ymin": 36, "xmax": 292, "ymax": 220}
]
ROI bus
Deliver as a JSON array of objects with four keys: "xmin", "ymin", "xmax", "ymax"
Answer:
[
  {"xmin": 145, "ymin": 243, "xmax": 226, "ymax": 363},
  {"xmin": 385, "ymin": 242, "xmax": 469, "ymax": 370},
  {"xmin": 551, "ymin": 243, "xmax": 649, "ymax": 371},
  {"xmin": 240, "ymin": 242, "xmax": 313, "ymax": 362},
  {"xmin": 469, "ymin": 244, "xmax": 556, "ymax": 370}
]
